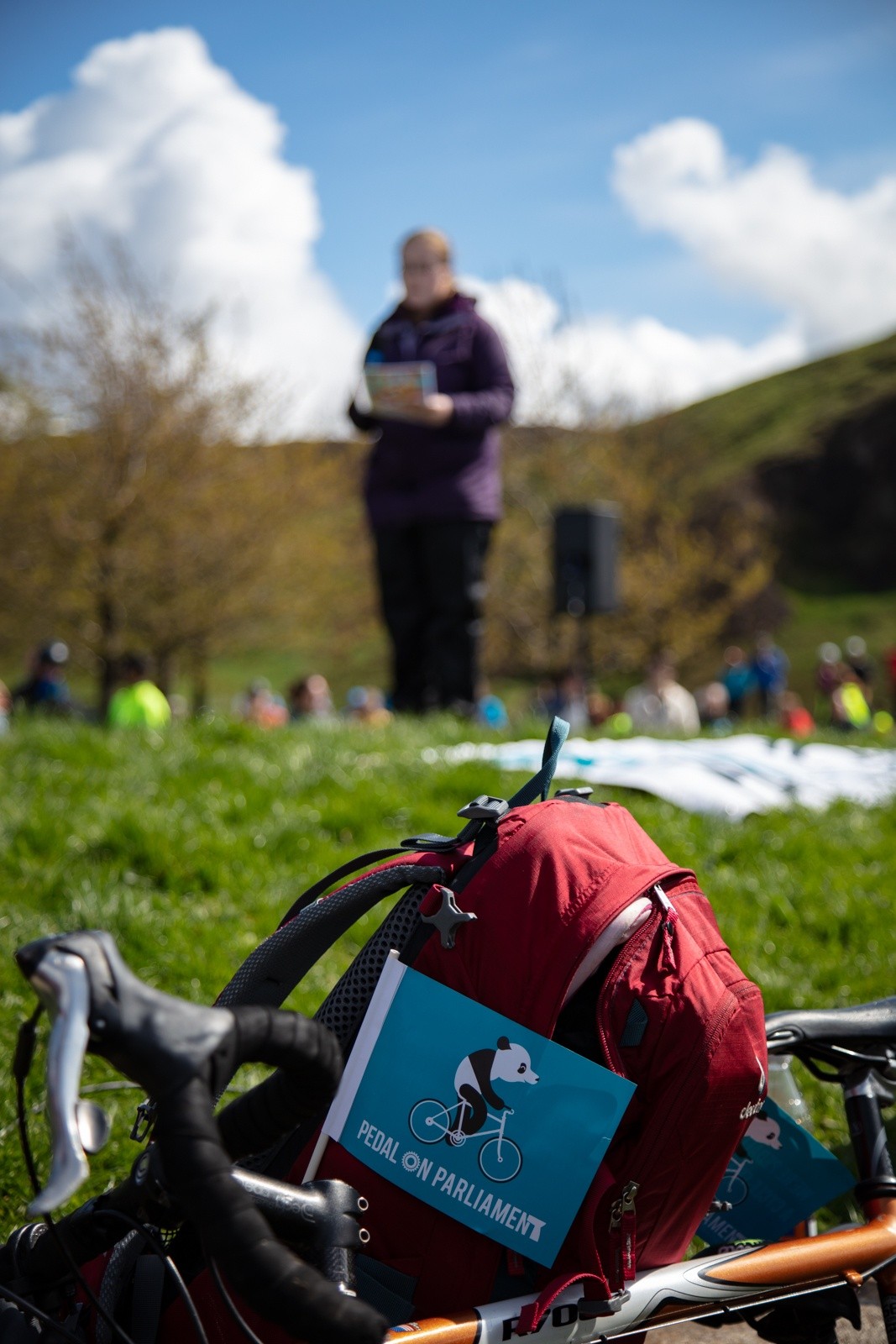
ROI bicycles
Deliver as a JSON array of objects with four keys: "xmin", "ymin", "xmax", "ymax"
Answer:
[{"xmin": 0, "ymin": 667, "xmax": 895, "ymax": 1344}]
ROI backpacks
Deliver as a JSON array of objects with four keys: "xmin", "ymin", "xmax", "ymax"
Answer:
[{"xmin": 51, "ymin": 714, "xmax": 767, "ymax": 1344}]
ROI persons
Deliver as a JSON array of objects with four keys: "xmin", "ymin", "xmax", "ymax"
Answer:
[
  {"xmin": 535, "ymin": 642, "xmax": 896, "ymax": 740},
  {"xmin": 0, "ymin": 640, "xmax": 383, "ymax": 737},
  {"xmin": 347, "ymin": 228, "xmax": 514, "ymax": 723}
]
